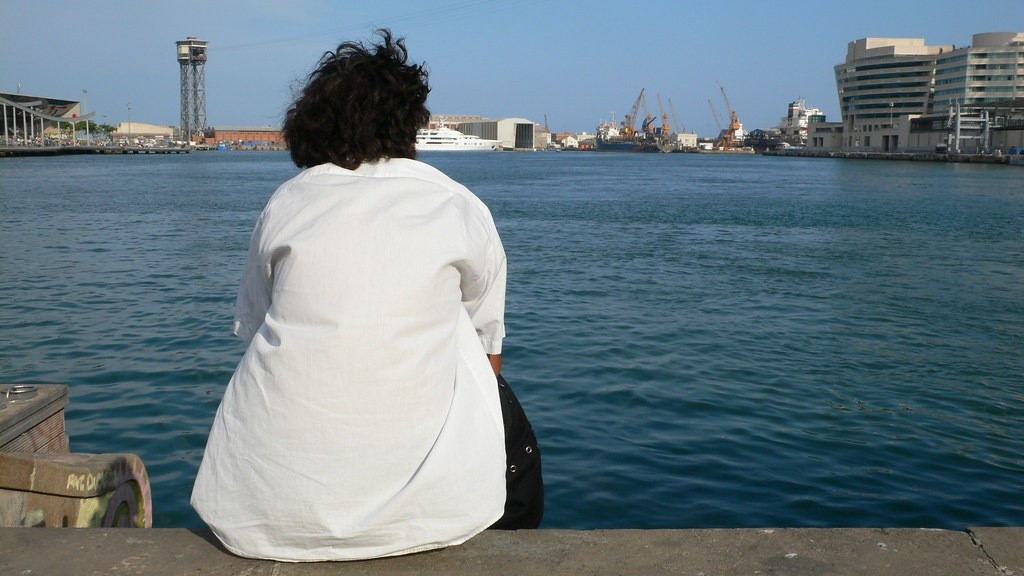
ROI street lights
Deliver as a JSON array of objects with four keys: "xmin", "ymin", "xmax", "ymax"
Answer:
[
  {"xmin": 889, "ymin": 100, "xmax": 894, "ymax": 127},
  {"xmin": 126, "ymin": 102, "xmax": 132, "ymax": 134}
]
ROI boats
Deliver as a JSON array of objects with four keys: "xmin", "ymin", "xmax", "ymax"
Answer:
[
  {"xmin": 596, "ymin": 111, "xmax": 678, "ymax": 154},
  {"xmin": 414, "ymin": 118, "xmax": 502, "ymax": 151}
]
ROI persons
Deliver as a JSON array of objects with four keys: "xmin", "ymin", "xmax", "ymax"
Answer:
[{"xmin": 190, "ymin": 28, "xmax": 547, "ymax": 563}]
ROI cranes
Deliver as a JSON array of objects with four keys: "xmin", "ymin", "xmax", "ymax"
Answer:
[
  {"xmin": 706, "ymin": 79, "xmax": 740, "ymax": 152},
  {"xmin": 619, "ymin": 88, "xmax": 683, "ymax": 148}
]
